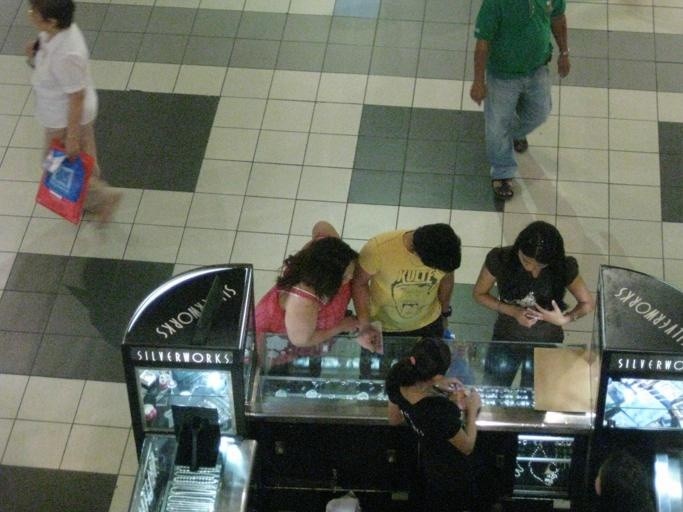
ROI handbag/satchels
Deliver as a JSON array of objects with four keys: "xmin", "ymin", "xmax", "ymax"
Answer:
[
  {"xmin": 26, "ymin": 48, "xmax": 38, "ymax": 70},
  {"xmin": 33, "ymin": 136, "xmax": 97, "ymax": 226}
]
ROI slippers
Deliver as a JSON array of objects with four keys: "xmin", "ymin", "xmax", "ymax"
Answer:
[{"xmin": 492, "ymin": 135, "xmax": 529, "ymax": 200}]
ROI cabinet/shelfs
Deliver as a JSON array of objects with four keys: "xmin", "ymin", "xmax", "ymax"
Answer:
[
  {"xmin": 122, "ymin": 263, "xmax": 259, "ymax": 460},
  {"xmin": 590, "ymin": 263, "xmax": 683, "ymax": 452}
]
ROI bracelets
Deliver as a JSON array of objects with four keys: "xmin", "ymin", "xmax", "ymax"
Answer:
[{"xmin": 559, "ymin": 48, "xmax": 570, "ymax": 56}]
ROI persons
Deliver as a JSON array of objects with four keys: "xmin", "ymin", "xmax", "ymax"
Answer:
[
  {"xmin": 469, "ymin": 1, "xmax": 571, "ymax": 199},
  {"xmin": 384, "ymin": 339, "xmax": 482, "ymax": 511},
  {"xmin": 251, "ymin": 221, "xmax": 361, "ymax": 370},
  {"xmin": 591, "ymin": 453, "xmax": 658, "ymax": 511},
  {"xmin": 22, "ymin": 1, "xmax": 125, "ymax": 230},
  {"xmin": 470, "ymin": 221, "xmax": 596, "ymax": 390},
  {"xmin": 350, "ymin": 223, "xmax": 461, "ymax": 378}
]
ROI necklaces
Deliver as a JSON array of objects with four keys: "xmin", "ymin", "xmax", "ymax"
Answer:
[{"xmin": 160, "ymin": 462, "xmax": 222, "ymax": 512}]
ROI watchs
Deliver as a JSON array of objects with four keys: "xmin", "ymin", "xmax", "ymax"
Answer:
[{"xmin": 441, "ymin": 305, "xmax": 453, "ymax": 317}]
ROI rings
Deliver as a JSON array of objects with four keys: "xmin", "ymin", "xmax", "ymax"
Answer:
[
  {"xmin": 475, "ymin": 384, "xmax": 537, "ymax": 409},
  {"xmin": 533, "ymin": 314, "xmax": 539, "ymax": 321}
]
